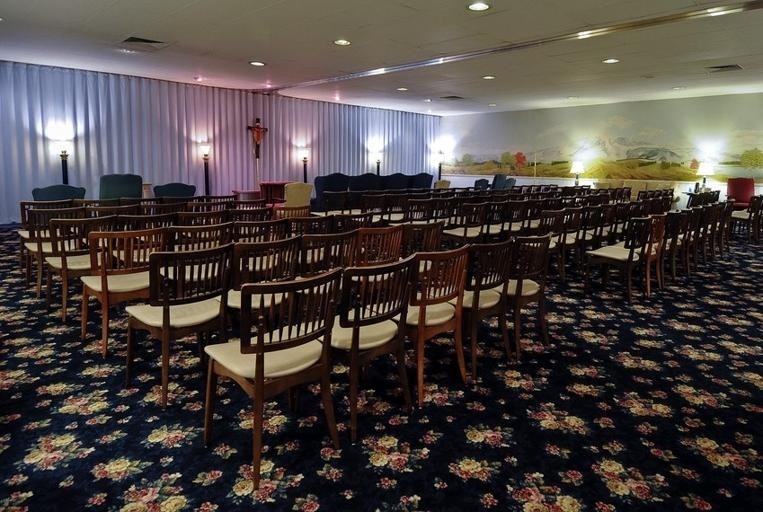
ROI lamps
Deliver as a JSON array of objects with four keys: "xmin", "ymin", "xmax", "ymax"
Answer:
[
  {"xmin": 694, "ymin": 161, "xmax": 715, "ymax": 192},
  {"xmin": 198, "ymin": 144, "xmax": 213, "ymax": 198},
  {"xmin": 435, "ymin": 153, "xmax": 445, "ymax": 181},
  {"xmin": 373, "ymin": 153, "xmax": 383, "ymax": 177},
  {"xmin": 569, "ymin": 160, "xmax": 584, "ymax": 186},
  {"xmin": 48, "ymin": 139, "xmax": 74, "ymax": 184},
  {"xmin": 298, "ymin": 145, "xmax": 312, "ymax": 184}
]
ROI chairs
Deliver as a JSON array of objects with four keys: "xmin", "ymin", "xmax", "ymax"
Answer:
[
  {"xmin": 11, "ymin": 171, "xmax": 404, "ymax": 406},
  {"xmin": 478, "ymin": 172, "xmax": 763, "ymax": 309},
  {"xmin": 200, "ymin": 267, "xmax": 342, "ymax": 491},
  {"xmin": 402, "ymin": 219, "xmax": 445, "ymax": 277},
  {"xmin": 366, "ymin": 242, "xmax": 471, "ymax": 410},
  {"xmin": 479, "ymin": 233, "xmax": 552, "ymax": 361},
  {"xmin": 386, "ymin": 184, "xmax": 476, "ymax": 246},
  {"xmin": 424, "ymin": 231, "xmax": 515, "ymax": 378},
  {"xmin": 293, "ymin": 252, "xmax": 417, "ymax": 442}
]
